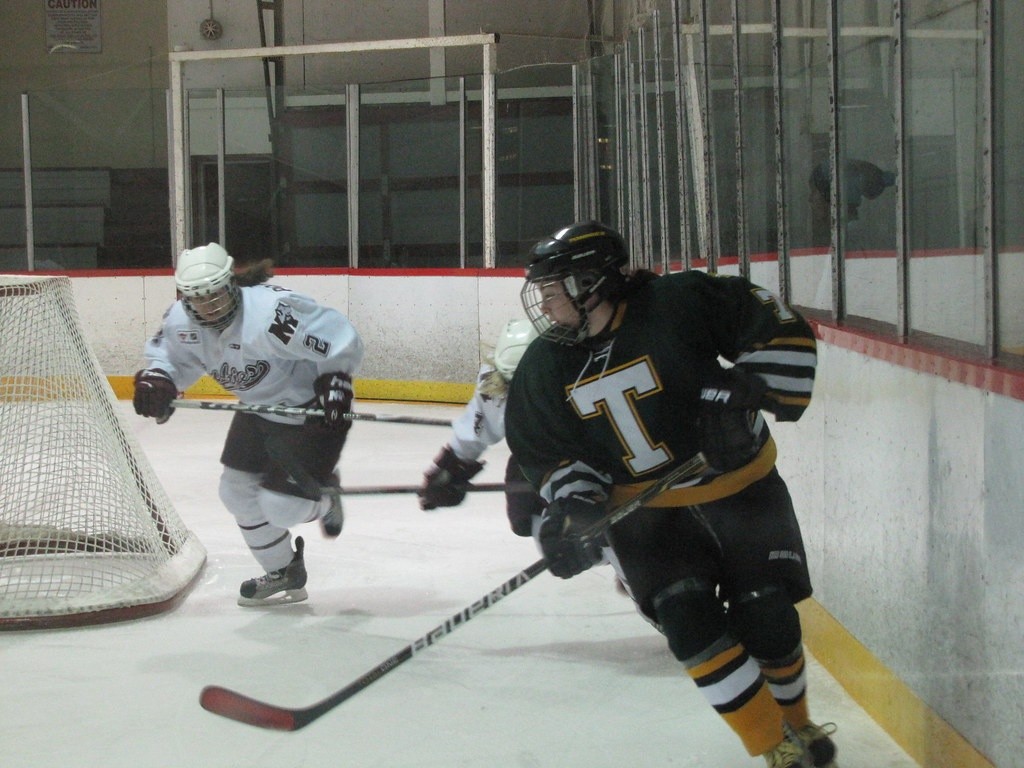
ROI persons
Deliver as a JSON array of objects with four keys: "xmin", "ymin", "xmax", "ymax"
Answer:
[
  {"xmin": 808, "ymin": 156, "xmax": 932, "ymax": 330},
  {"xmin": 418, "ymin": 314, "xmax": 669, "ymax": 638},
  {"xmin": 504, "ymin": 221, "xmax": 839, "ymax": 768},
  {"xmin": 133, "ymin": 241, "xmax": 364, "ymax": 608}
]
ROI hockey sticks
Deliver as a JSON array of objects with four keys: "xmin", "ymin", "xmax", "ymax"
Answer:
[
  {"xmin": 262, "ymin": 431, "xmax": 506, "ymax": 499},
  {"xmin": 167, "ymin": 397, "xmax": 451, "ymax": 429},
  {"xmin": 195, "ymin": 449, "xmax": 714, "ymax": 735}
]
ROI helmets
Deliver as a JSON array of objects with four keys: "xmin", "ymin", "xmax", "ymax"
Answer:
[
  {"xmin": 520, "ymin": 221, "xmax": 633, "ymax": 349},
  {"xmin": 175, "ymin": 242, "xmax": 238, "ymax": 332},
  {"xmin": 494, "ymin": 317, "xmax": 553, "ymax": 383}
]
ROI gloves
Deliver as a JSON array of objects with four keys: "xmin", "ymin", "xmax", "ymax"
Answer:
[
  {"xmin": 419, "ymin": 443, "xmax": 485, "ymax": 510},
  {"xmin": 539, "ymin": 492, "xmax": 611, "ymax": 582},
  {"xmin": 690, "ymin": 368, "xmax": 767, "ymax": 471},
  {"xmin": 303, "ymin": 371, "xmax": 354, "ymax": 441},
  {"xmin": 132, "ymin": 368, "xmax": 179, "ymax": 427}
]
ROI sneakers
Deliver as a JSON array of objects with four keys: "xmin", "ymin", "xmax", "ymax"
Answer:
[
  {"xmin": 794, "ymin": 720, "xmax": 835, "ymax": 768},
  {"xmin": 238, "ymin": 534, "xmax": 307, "ymax": 607},
  {"xmin": 318, "ymin": 472, "xmax": 342, "ymax": 538},
  {"xmin": 764, "ymin": 722, "xmax": 815, "ymax": 768}
]
